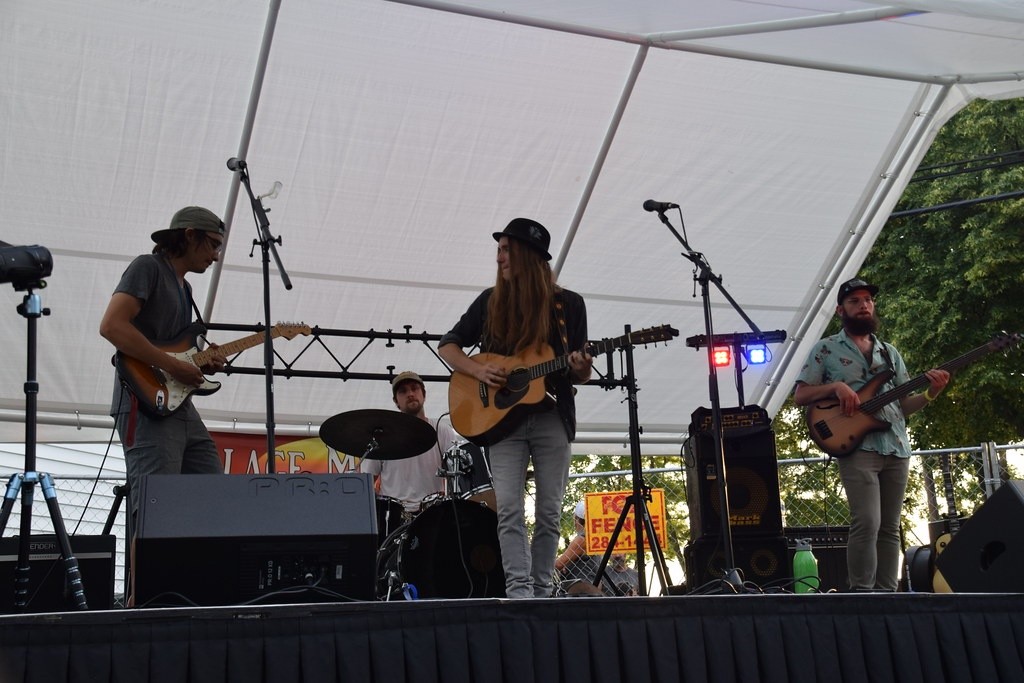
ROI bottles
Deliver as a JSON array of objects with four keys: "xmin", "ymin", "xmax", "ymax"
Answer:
[{"xmin": 793, "ymin": 538, "xmax": 819, "ymax": 594}]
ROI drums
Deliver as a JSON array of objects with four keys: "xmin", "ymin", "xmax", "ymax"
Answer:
[
  {"xmin": 375, "ymin": 494, "xmax": 407, "ymax": 549},
  {"xmin": 442, "ymin": 438, "xmax": 499, "ymax": 513},
  {"xmin": 377, "ymin": 498, "xmax": 508, "ymax": 601},
  {"xmin": 419, "ymin": 491, "xmax": 453, "ymax": 513}
]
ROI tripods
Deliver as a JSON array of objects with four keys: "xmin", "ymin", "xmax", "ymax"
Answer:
[
  {"xmin": 0, "ymin": 295, "xmax": 89, "ymax": 613},
  {"xmin": 591, "ymin": 324, "xmax": 674, "ymax": 596}
]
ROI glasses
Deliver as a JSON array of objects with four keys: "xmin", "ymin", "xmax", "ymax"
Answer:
[
  {"xmin": 205, "ymin": 234, "xmax": 222, "ymax": 255},
  {"xmin": 842, "ymin": 296, "xmax": 876, "ymax": 305}
]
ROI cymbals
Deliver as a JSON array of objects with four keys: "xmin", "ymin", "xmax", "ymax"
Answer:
[{"xmin": 319, "ymin": 408, "xmax": 438, "ymax": 461}]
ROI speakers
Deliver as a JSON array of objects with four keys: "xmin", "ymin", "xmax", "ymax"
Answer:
[
  {"xmin": 683, "ymin": 535, "xmax": 794, "ymax": 594},
  {"xmin": 0, "ymin": 535, "xmax": 117, "ymax": 611},
  {"xmin": 129, "ymin": 471, "xmax": 379, "ymax": 609},
  {"xmin": 935, "ymin": 478, "xmax": 1024, "ymax": 594},
  {"xmin": 683, "ymin": 432, "xmax": 785, "ymax": 534},
  {"xmin": 786, "ymin": 526, "xmax": 854, "ymax": 592}
]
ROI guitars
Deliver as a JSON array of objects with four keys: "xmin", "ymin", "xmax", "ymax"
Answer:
[
  {"xmin": 931, "ymin": 451, "xmax": 961, "ymax": 593},
  {"xmin": 114, "ymin": 319, "xmax": 311, "ymax": 422},
  {"xmin": 447, "ymin": 323, "xmax": 680, "ymax": 448},
  {"xmin": 805, "ymin": 329, "xmax": 1018, "ymax": 459}
]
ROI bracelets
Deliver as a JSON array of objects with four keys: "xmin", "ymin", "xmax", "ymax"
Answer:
[{"xmin": 925, "ymin": 388, "xmax": 936, "ymax": 401}]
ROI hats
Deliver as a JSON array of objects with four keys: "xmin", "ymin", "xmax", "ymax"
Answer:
[
  {"xmin": 574, "ymin": 498, "xmax": 585, "ymax": 520},
  {"xmin": 838, "ymin": 277, "xmax": 879, "ymax": 305},
  {"xmin": 151, "ymin": 205, "xmax": 226, "ymax": 249},
  {"xmin": 392, "ymin": 371, "xmax": 424, "ymax": 394},
  {"xmin": 492, "ymin": 218, "xmax": 552, "ymax": 261}
]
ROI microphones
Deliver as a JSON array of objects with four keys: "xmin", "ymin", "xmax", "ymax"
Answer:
[
  {"xmin": 644, "ymin": 200, "xmax": 679, "ymax": 214},
  {"xmin": 229, "ymin": 158, "xmax": 249, "ymax": 171}
]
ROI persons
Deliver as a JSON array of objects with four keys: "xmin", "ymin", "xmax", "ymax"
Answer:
[
  {"xmin": 556, "ymin": 501, "xmax": 640, "ymax": 596},
  {"xmin": 793, "ymin": 277, "xmax": 950, "ymax": 593},
  {"xmin": 437, "ymin": 217, "xmax": 593, "ymax": 598},
  {"xmin": 99, "ymin": 205, "xmax": 227, "ymax": 604},
  {"xmin": 360, "ymin": 370, "xmax": 461, "ymax": 514}
]
